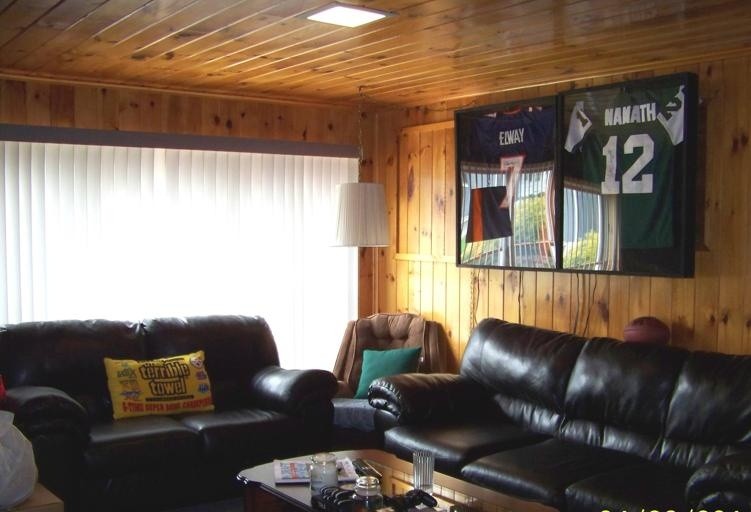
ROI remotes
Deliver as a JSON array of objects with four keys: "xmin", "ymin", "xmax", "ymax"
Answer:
[{"xmin": 353, "ymin": 457, "xmax": 383, "ymax": 479}]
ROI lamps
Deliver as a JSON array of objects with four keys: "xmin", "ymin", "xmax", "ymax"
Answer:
[{"xmin": 330, "ymin": 86, "xmax": 391, "ymax": 247}]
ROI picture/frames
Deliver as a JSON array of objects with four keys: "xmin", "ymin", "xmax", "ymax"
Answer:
[
  {"xmin": 453, "ymin": 95, "xmax": 560, "ymax": 272},
  {"xmin": 559, "ymin": 72, "xmax": 700, "ymax": 278}
]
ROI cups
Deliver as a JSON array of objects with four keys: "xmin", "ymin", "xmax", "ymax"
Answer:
[{"xmin": 413, "ymin": 450, "xmax": 434, "ymax": 496}]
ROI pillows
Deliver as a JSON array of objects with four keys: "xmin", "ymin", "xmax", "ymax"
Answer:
[
  {"xmin": 355, "ymin": 345, "xmax": 421, "ymax": 399},
  {"xmin": 104, "ymin": 350, "xmax": 215, "ymax": 419}
]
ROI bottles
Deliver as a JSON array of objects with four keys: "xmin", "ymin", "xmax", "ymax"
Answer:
[
  {"xmin": 311, "ymin": 452, "xmax": 339, "ymax": 494},
  {"xmin": 352, "ymin": 476, "xmax": 383, "ymax": 512}
]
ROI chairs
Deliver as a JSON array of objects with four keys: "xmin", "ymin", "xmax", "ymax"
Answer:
[
  {"xmin": 0, "ymin": 316, "xmax": 338, "ymax": 512},
  {"xmin": 331, "ymin": 312, "xmax": 456, "ymax": 448}
]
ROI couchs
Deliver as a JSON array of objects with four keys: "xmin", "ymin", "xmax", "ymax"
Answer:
[{"xmin": 368, "ymin": 318, "xmax": 751, "ymax": 512}]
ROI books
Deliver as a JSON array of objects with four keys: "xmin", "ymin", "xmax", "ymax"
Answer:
[{"xmin": 272, "ymin": 457, "xmax": 360, "ymax": 484}]
